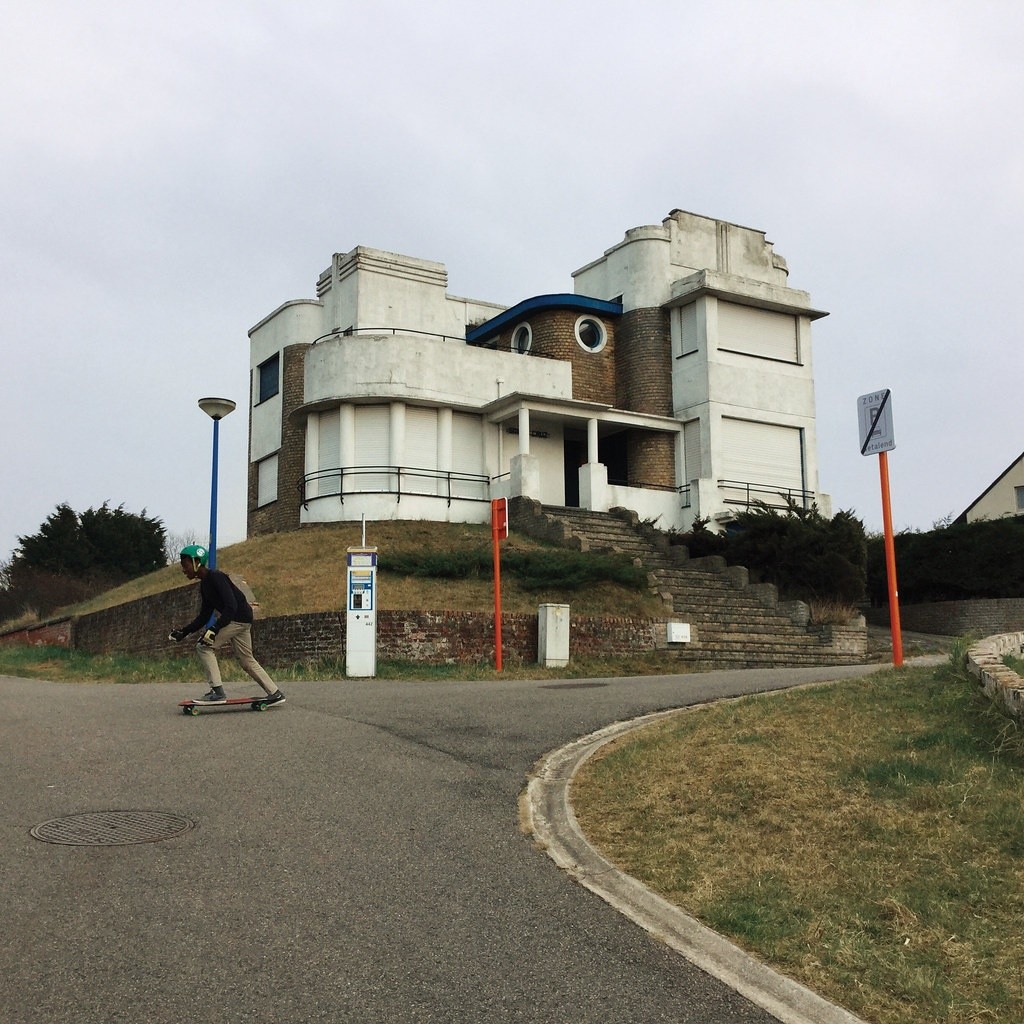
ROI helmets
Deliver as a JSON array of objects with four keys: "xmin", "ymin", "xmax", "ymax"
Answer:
[{"xmin": 180, "ymin": 545, "xmax": 209, "ymax": 566}]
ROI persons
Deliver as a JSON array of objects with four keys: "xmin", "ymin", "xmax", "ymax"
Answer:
[{"xmin": 169, "ymin": 545, "xmax": 285, "ymax": 702}]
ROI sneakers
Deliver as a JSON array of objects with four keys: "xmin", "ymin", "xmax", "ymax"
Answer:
[
  {"xmin": 191, "ymin": 689, "xmax": 227, "ymax": 705},
  {"xmin": 253, "ymin": 690, "xmax": 286, "ymax": 708}
]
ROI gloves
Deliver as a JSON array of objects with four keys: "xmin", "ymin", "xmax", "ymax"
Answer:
[
  {"xmin": 197, "ymin": 626, "xmax": 217, "ymax": 646},
  {"xmin": 168, "ymin": 627, "xmax": 186, "ymax": 644}
]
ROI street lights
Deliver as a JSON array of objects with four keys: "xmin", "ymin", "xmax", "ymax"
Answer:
[{"xmin": 196, "ymin": 396, "xmax": 235, "ymax": 664}]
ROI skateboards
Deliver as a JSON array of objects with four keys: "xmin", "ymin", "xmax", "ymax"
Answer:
[{"xmin": 177, "ymin": 693, "xmax": 282, "ymax": 716}]
